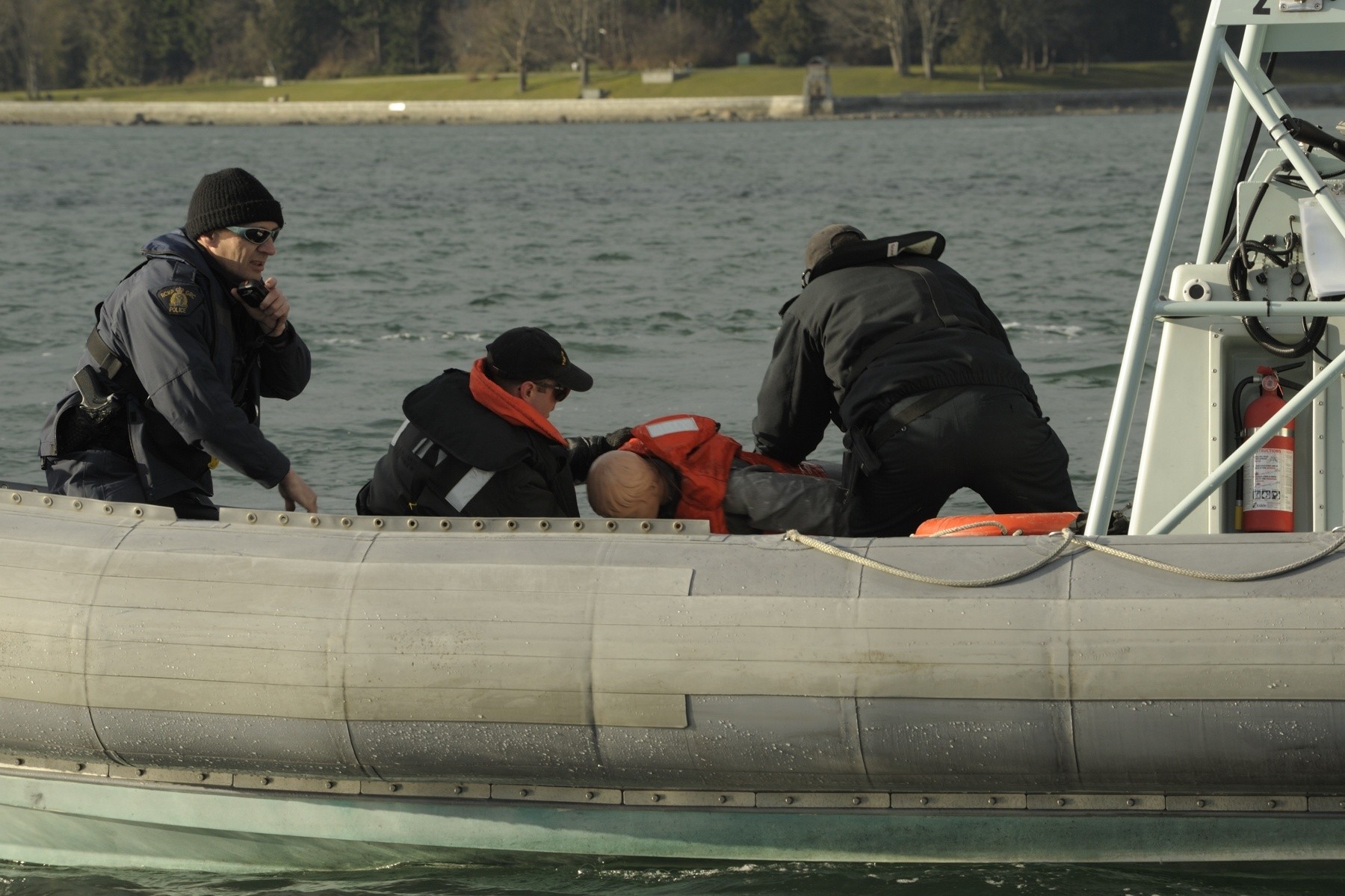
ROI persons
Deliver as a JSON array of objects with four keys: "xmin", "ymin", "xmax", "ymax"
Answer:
[
  {"xmin": 586, "ymin": 414, "xmax": 845, "ymax": 535},
  {"xmin": 751, "ymin": 224, "xmax": 1081, "ymax": 539},
  {"xmin": 356, "ymin": 327, "xmax": 633, "ymax": 517},
  {"xmin": 38, "ymin": 169, "xmax": 317, "ymax": 523}
]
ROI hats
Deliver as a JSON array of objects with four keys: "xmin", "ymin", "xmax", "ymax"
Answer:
[
  {"xmin": 485, "ymin": 327, "xmax": 593, "ymax": 392},
  {"xmin": 184, "ymin": 167, "xmax": 283, "ymax": 242},
  {"xmin": 806, "ymin": 223, "xmax": 866, "ymax": 272}
]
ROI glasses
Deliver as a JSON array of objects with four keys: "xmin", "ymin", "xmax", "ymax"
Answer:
[
  {"xmin": 224, "ymin": 224, "xmax": 281, "ymax": 244},
  {"xmin": 518, "ymin": 381, "xmax": 571, "ymax": 402}
]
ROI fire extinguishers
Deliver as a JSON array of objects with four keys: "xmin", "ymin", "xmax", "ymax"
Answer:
[{"xmin": 1232, "ymin": 361, "xmax": 1304, "ymax": 533}]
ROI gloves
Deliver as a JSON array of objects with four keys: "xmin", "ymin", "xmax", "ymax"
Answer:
[{"xmin": 589, "ymin": 425, "xmax": 634, "ymax": 455}]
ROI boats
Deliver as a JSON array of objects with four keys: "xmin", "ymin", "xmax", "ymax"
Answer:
[{"xmin": 0, "ymin": 1, "xmax": 1345, "ymax": 890}]
